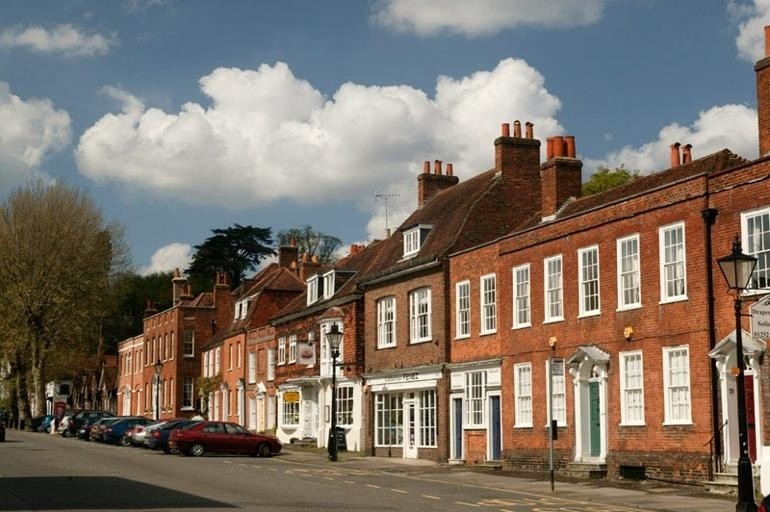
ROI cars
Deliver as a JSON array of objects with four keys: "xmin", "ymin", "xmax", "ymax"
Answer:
[
  {"xmin": 33, "ymin": 406, "xmax": 283, "ymax": 461},
  {"xmin": 1, "ymin": 420, "xmax": 6, "ymax": 442}
]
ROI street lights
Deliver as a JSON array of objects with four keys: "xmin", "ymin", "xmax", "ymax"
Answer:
[
  {"xmin": 716, "ymin": 231, "xmax": 762, "ymax": 511},
  {"xmin": 325, "ymin": 320, "xmax": 347, "ymax": 461},
  {"xmin": 152, "ymin": 359, "xmax": 162, "ymax": 420}
]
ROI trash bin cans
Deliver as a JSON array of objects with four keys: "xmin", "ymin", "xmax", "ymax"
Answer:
[
  {"xmin": 329, "ymin": 428, "xmax": 346, "ymax": 452},
  {"xmin": 620, "ymin": 464, "xmax": 646, "ymax": 480}
]
